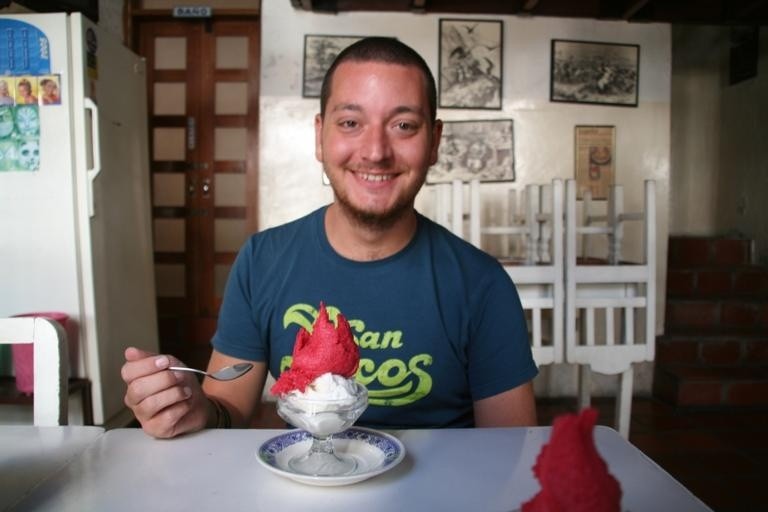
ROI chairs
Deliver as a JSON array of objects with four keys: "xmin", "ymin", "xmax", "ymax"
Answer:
[
  {"xmin": 413, "ymin": 175, "xmax": 657, "ymax": 374},
  {"xmin": 0, "ymin": 316, "xmax": 68, "ymax": 424}
]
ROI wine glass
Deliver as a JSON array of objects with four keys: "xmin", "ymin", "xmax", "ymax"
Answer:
[{"xmin": 277, "ymin": 382, "xmax": 369, "ymax": 478}]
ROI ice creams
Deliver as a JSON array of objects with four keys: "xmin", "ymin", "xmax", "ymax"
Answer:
[
  {"xmin": 517, "ymin": 405, "xmax": 623, "ymax": 512},
  {"xmin": 268, "ymin": 300, "xmax": 368, "ymax": 438}
]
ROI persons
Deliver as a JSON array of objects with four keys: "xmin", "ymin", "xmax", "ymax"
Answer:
[
  {"xmin": 17, "ymin": 78, "xmax": 38, "ymax": 104},
  {"xmin": 0, "ymin": 79, "xmax": 14, "ymax": 105},
  {"xmin": 40, "ymin": 79, "xmax": 60, "ymax": 104},
  {"xmin": 121, "ymin": 37, "xmax": 538, "ymax": 439}
]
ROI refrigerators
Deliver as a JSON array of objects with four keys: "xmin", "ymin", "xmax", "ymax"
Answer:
[{"xmin": 2, "ymin": 10, "xmax": 163, "ymax": 427}]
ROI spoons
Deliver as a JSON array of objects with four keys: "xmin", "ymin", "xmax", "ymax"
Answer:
[{"xmin": 166, "ymin": 363, "xmax": 254, "ymax": 380}]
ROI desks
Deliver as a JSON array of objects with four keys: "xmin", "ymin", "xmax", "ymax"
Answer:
[
  {"xmin": 490, "ymin": 257, "xmax": 647, "ymax": 443},
  {"xmin": 0, "ymin": 375, "xmax": 94, "ymax": 426},
  {"xmin": 0, "ymin": 424, "xmax": 711, "ymax": 512}
]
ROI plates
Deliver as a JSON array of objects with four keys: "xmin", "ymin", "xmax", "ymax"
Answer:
[{"xmin": 256, "ymin": 426, "xmax": 406, "ymax": 488}]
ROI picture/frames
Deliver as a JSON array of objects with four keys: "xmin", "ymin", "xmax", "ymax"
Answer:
[
  {"xmin": 548, "ymin": 38, "xmax": 640, "ymax": 107},
  {"xmin": 437, "ymin": 17, "xmax": 504, "ymax": 110},
  {"xmin": 425, "ymin": 118, "xmax": 515, "ymax": 185},
  {"xmin": 301, "ymin": 33, "xmax": 368, "ymax": 98}
]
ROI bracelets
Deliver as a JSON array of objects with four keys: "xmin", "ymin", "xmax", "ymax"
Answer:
[{"xmin": 207, "ymin": 394, "xmax": 232, "ymax": 428}]
